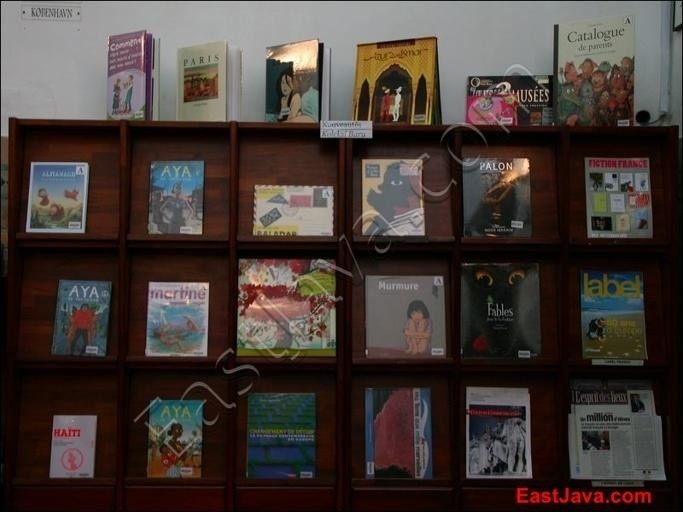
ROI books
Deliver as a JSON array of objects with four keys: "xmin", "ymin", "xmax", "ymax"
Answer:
[{"xmin": 26, "ymin": 12, "xmax": 667, "ymax": 487}]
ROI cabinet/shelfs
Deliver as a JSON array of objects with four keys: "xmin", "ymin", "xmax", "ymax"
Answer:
[
  {"xmin": 342, "ymin": 120, "xmax": 682, "ymax": 511},
  {"xmin": 0, "ymin": 113, "xmax": 344, "ymax": 512}
]
